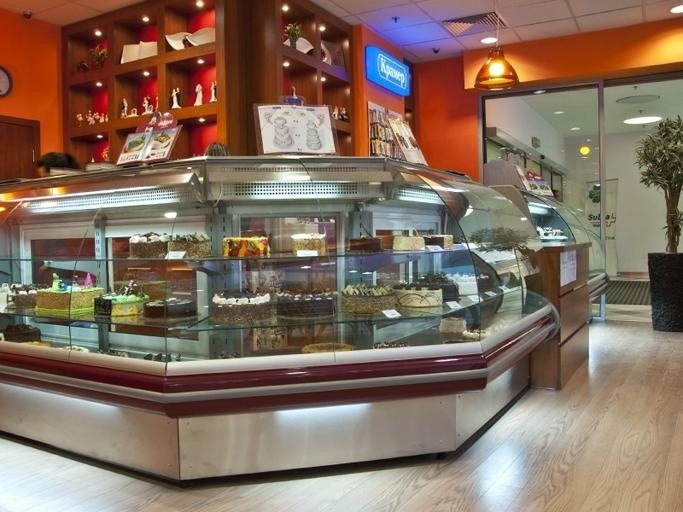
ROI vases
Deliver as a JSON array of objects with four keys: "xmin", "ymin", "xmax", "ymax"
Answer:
[{"xmin": 289, "ymin": 36, "xmax": 297, "ymax": 50}]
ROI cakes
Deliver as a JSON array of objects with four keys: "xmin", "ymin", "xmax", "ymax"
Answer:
[
  {"xmin": 0, "ymin": 230, "xmax": 527, "ymax": 363},
  {"xmin": 274, "ymin": 117, "xmax": 292, "ymax": 146},
  {"xmin": 306, "ymin": 120, "xmax": 321, "ymax": 150}
]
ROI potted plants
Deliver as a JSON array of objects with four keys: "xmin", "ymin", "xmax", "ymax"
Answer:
[{"xmin": 637, "ymin": 111, "xmax": 683, "ymax": 335}]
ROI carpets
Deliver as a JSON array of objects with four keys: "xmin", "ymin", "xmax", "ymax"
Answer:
[{"xmin": 591, "ymin": 274, "xmax": 658, "ymax": 309}]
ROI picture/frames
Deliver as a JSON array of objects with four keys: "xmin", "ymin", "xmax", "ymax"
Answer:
[{"xmin": 0, "ymin": 66, "xmax": 14, "ymax": 98}]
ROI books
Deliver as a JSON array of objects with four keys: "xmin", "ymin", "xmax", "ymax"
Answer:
[
  {"xmin": 49, "ymin": 166, "xmax": 83, "ymax": 176},
  {"xmin": 83, "ymin": 160, "xmax": 119, "ymax": 171},
  {"xmin": 117, "ymin": 124, "xmax": 183, "ymax": 166}
]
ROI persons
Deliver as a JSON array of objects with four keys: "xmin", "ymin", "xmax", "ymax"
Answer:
[
  {"xmin": 33, "ymin": 150, "xmax": 93, "ymax": 284},
  {"xmin": 203, "ymin": 140, "xmax": 251, "ymax": 237},
  {"xmin": 120, "ymin": 95, "xmax": 158, "ymax": 117},
  {"xmin": 76, "ymin": 110, "xmax": 108, "ymax": 127},
  {"xmin": 170, "ymin": 80, "xmax": 217, "ymax": 108}
]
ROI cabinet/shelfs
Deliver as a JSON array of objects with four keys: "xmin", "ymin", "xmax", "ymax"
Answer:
[
  {"xmin": 246, "ymin": 0, "xmax": 357, "ymax": 155},
  {"xmin": 0, "ymin": 154, "xmax": 561, "ymax": 491},
  {"xmin": 57, "ymin": 1, "xmax": 244, "ymax": 176},
  {"xmin": 545, "ymin": 195, "xmax": 612, "ymax": 303},
  {"xmin": 485, "ymin": 181, "xmax": 593, "ymax": 392}
]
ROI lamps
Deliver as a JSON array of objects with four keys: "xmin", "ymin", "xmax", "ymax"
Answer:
[{"xmin": 472, "ymin": 2, "xmax": 522, "ymax": 95}]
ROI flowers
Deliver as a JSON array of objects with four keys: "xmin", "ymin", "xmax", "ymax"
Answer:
[{"xmin": 283, "ymin": 20, "xmax": 302, "ymax": 43}]
